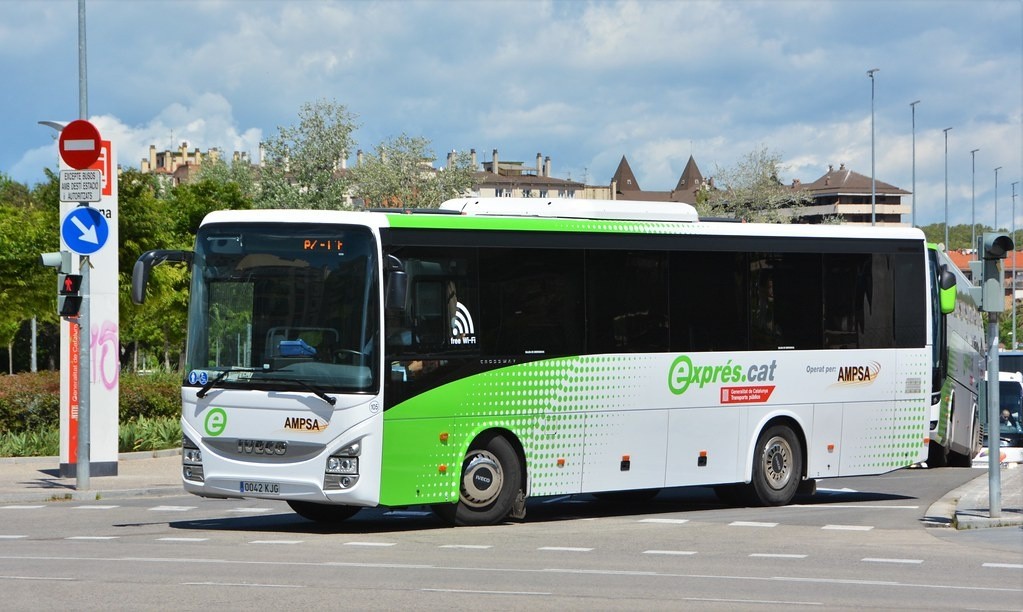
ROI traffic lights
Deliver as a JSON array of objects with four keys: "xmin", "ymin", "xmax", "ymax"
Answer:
[
  {"xmin": 36, "ymin": 250, "xmax": 67, "ymax": 272},
  {"xmin": 55, "ymin": 274, "xmax": 83, "ymax": 317},
  {"xmin": 980, "ymin": 232, "xmax": 1015, "ymax": 261}
]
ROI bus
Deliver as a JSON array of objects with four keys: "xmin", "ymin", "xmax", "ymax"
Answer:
[
  {"xmin": 971, "ymin": 351, "xmax": 1023, "ymax": 468},
  {"xmin": 130, "ymin": 196, "xmax": 934, "ymax": 528},
  {"xmin": 924, "ymin": 241, "xmax": 985, "ymax": 468}
]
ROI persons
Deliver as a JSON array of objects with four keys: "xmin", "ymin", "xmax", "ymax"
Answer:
[
  {"xmin": 999, "ymin": 408, "xmax": 1013, "ymax": 430},
  {"xmin": 357, "ymin": 307, "xmax": 424, "ymax": 374}
]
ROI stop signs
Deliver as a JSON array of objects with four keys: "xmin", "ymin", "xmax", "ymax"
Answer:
[{"xmin": 58, "ymin": 118, "xmax": 103, "ymax": 171}]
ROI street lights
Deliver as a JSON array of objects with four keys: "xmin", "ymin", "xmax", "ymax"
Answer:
[
  {"xmin": 866, "ymin": 68, "xmax": 880, "ymax": 226},
  {"xmin": 1011, "ymin": 181, "xmax": 1021, "ymax": 352},
  {"xmin": 993, "ymin": 165, "xmax": 1002, "ymax": 233},
  {"xmin": 908, "ymin": 100, "xmax": 923, "ymax": 227},
  {"xmin": 943, "ymin": 127, "xmax": 955, "ymax": 253},
  {"xmin": 969, "ymin": 149, "xmax": 980, "ymax": 263}
]
